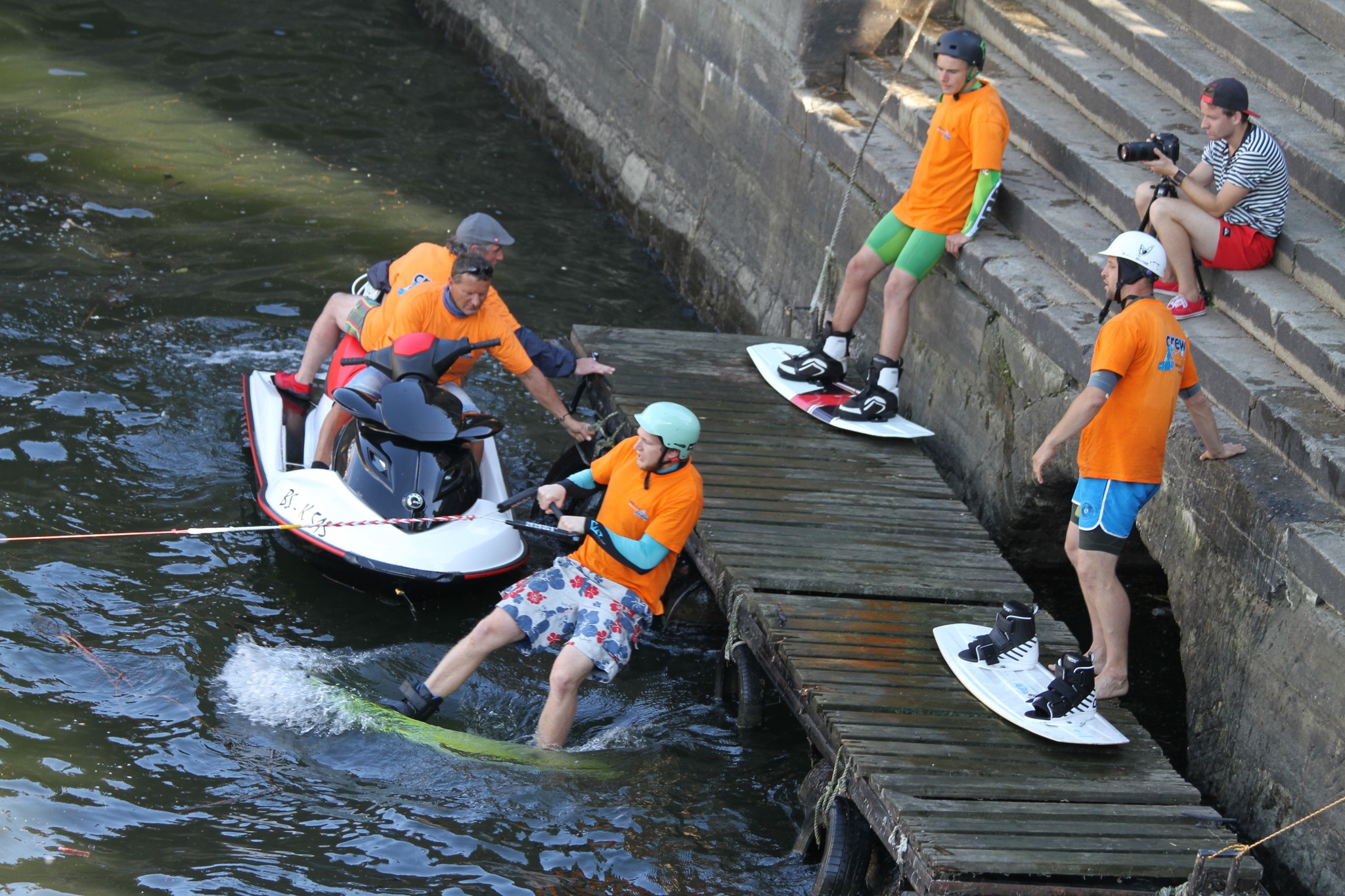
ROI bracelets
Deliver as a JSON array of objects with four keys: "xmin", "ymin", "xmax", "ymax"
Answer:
[
  {"xmin": 552, "ymin": 414, "xmax": 573, "ymax": 425},
  {"xmin": 1169, "ymin": 168, "xmax": 1188, "ymax": 187}
]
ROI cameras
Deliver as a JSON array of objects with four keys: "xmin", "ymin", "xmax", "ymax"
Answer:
[{"xmin": 1117, "ymin": 132, "xmax": 1179, "ymax": 165}]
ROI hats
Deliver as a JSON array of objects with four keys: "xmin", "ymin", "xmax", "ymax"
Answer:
[
  {"xmin": 455, "ymin": 212, "xmax": 514, "ymax": 245},
  {"xmin": 1201, "ymin": 77, "xmax": 1261, "ymax": 118}
]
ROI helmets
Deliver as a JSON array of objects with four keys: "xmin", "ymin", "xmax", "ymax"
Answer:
[
  {"xmin": 634, "ymin": 401, "xmax": 700, "ymax": 460},
  {"xmin": 933, "ymin": 29, "xmax": 986, "ymax": 72},
  {"xmin": 1098, "ymin": 231, "xmax": 1166, "ymax": 279}
]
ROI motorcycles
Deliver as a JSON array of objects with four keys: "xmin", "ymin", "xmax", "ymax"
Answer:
[{"xmin": 241, "ymin": 334, "xmax": 529, "ymax": 587}]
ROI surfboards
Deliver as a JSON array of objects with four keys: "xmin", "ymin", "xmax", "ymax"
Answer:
[
  {"xmin": 745, "ymin": 343, "xmax": 935, "ymax": 438},
  {"xmin": 932, "ymin": 623, "xmax": 1130, "ymax": 745}
]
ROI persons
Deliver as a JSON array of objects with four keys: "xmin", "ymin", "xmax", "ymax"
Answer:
[
  {"xmin": 777, "ymin": 30, "xmax": 1010, "ymax": 422},
  {"xmin": 1032, "ymin": 231, "xmax": 1246, "ymax": 699},
  {"xmin": 1135, "ymin": 77, "xmax": 1289, "ymax": 320},
  {"xmin": 385, "ymin": 402, "xmax": 703, "ymax": 752},
  {"xmin": 273, "ymin": 212, "xmax": 615, "ymax": 470}
]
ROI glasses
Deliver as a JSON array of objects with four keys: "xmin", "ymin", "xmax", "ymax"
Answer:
[{"xmin": 451, "ymin": 267, "xmax": 494, "ymax": 277}]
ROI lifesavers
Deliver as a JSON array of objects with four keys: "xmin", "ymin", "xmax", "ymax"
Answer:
[
  {"xmin": 790, "ymin": 793, "xmax": 873, "ymax": 896},
  {"xmin": 716, "ymin": 639, "xmax": 766, "ymax": 729},
  {"xmin": 529, "ymin": 438, "xmax": 606, "ymax": 549}
]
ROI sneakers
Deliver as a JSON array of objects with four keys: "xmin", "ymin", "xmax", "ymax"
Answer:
[
  {"xmin": 1024, "ymin": 652, "xmax": 1097, "ymax": 726},
  {"xmin": 838, "ymin": 353, "xmax": 903, "ymax": 422},
  {"xmin": 1166, "ymin": 295, "xmax": 1206, "ymax": 320},
  {"xmin": 777, "ymin": 320, "xmax": 856, "ymax": 382},
  {"xmin": 274, "ymin": 371, "xmax": 313, "ymax": 400},
  {"xmin": 1153, "ymin": 278, "xmax": 1179, "ymax": 296},
  {"xmin": 958, "ymin": 600, "xmax": 1039, "ymax": 672},
  {"xmin": 377, "ymin": 680, "xmax": 443, "ymax": 722}
]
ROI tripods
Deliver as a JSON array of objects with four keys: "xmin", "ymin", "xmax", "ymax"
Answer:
[{"xmin": 1098, "ymin": 175, "xmax": 1209, "ymax": 324}]
